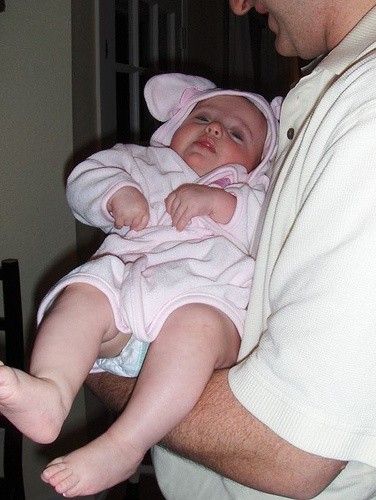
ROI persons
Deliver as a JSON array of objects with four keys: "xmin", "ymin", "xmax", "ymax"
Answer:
[
  {"xmin": 1, "ymin": 68, "xmax": 284, "ymax": 498},
  {"xmin": 83, "ymin": 0, "xmax": 375, "ymax": 500}
]
